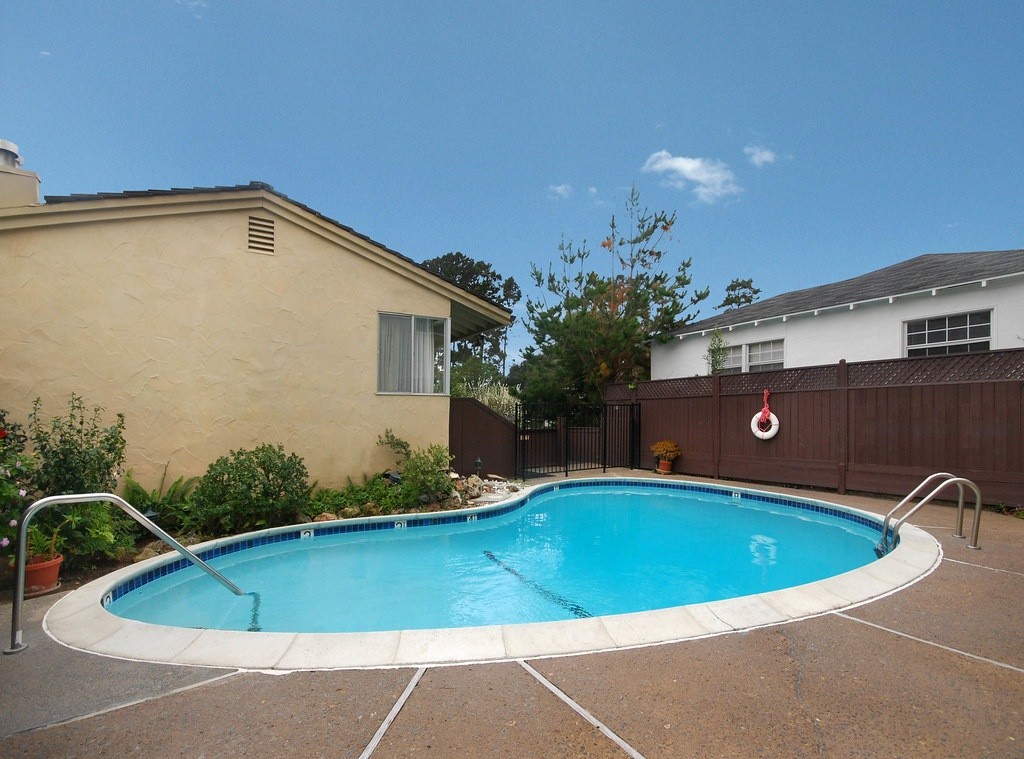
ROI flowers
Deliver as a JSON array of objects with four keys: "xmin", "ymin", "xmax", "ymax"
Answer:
[
  {"xmin": 650, "ymin": 441, "xmax": 681, "ymax": 461},
  {"xmin": 0, "ymin": 409, "xmax": 82, "ymax": 568}
]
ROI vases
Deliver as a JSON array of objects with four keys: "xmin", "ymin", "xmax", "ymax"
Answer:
[
  {"xmin": 24, "ymin": 554, "xmax": 63, "ymax": 594},
  {"xmin": 658, "ymin": 460, "xmax": 673, "ymax": 471}
]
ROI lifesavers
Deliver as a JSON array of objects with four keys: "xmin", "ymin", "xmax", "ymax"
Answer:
[{"xmin": 750, "ymin": 410, "xmax": 780, "ymax": 440}]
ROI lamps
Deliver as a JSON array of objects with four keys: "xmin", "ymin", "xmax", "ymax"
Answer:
[
  {"xmin": 389, "ymin": 471, "xmax": 401, "ymax": 485},
  {"xmin": 144, "ymin": 508, "xmax": 160, "ymax": 523},
  {"xmin": 475, "ymin": 457, "xmax": 482, "ymax": 467}
]
